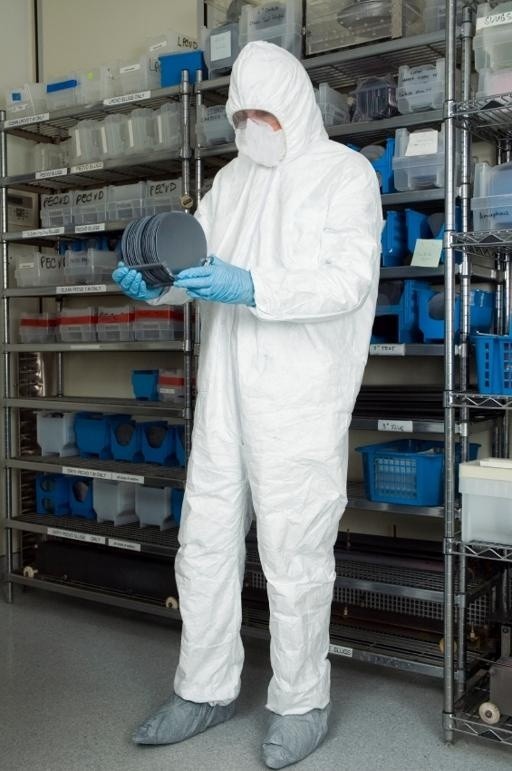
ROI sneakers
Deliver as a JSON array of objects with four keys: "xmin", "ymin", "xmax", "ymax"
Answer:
[
  {"xmin": 131, "ymin": 685, "xmax": 240, "ymax": 752},
  {"xmin": 263, "ymin": 705, "xmax": 328, "ymax": 771}
]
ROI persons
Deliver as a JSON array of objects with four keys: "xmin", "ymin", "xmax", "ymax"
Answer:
[{"xmin": 110, "ymin": 39, "xmax": 386, "ymax": 770}]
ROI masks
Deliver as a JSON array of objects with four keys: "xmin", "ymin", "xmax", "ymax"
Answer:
[{"xmin": 233, "ymin": 121, "xmax": 286, "ymax": 171}]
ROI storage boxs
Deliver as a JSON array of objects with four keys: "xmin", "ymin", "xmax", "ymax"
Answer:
[{"xmin": 5, "ymin": 42, "xmax": 207, "ymax": 121}]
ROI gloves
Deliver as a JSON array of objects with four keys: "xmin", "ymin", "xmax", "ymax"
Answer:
[
  {"xmin": 175, "ymin": 256, "xmax": 256, "ymax": 310},
  {"xmin": 113, "ymin": 258, "xmax": 168, "ymax": 302}
]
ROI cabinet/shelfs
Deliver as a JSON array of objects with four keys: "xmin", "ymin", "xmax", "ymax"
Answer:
[
  {"xmin": 1, "ymin": 63, "xmax": 192, "ymax": 624},
  {"xmin": 193, "ymin": 2, "xmax": 512, "ymax": 750}
]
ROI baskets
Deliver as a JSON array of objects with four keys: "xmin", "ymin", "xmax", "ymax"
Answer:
[
  {"xmin": 352, "ymin": 434, "xmax": 483, "ymax": 508},
  {"xmin": 466, "ymin": 324, "xmax": 511, "ymax": 398}
]
